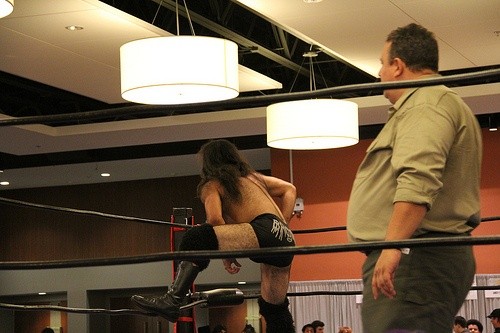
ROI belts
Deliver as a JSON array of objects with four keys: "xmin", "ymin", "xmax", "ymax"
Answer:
[{"xmin": 414, "ymin": 232, "xmax": 463, "ymax": 238}]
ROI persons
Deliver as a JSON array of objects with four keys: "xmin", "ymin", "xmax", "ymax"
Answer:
[
  {"xmin": 346, "ymin": 24, "xmax": 481, "ymax": 333},
  {"xmin": 338, "ymin": 327, "xmax": 352, "ymax": 333},
  {"xmin": 41, "ymin": 328, "xmax": 54, "ymax": 333},
  {"xmin": 131, "ymin": 139, "xmax": 296, "ymax": 333},
  {"xmin": 454, "ymin": 316, "xmax": 470, "ymax": 333},
  {"xmin": 467, "ymin": 319, "xmax": 484, "ymax": 333},
  {"xmin": 302, "ymin": 324, "xmax": 316, "ymax": 333},
  {"xmin": 312, "ymin": 321, "xmax": 325, "ymax": 333},
  {"xmin": 486, "ymin": 308, "xmax": 500, "ymax": 333}
]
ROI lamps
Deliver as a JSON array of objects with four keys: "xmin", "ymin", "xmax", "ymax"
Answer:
[
  {"xmin": 119, "ymin": 0, "xmax": 239, "ymax": 105},
  {"xmin": 0, "ymin": 0, "xmax": 15, "ymax": 19},
  {"xmin": 266, "ymin": 52, "xmax": 359, "ymax": 150}
]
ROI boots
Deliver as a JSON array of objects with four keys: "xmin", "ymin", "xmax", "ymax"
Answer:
[{"xmin": 130, "ymin": 260, "xmax": 200, "ymax": 323}]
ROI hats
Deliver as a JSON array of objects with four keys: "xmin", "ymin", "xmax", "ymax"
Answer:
[{"xmin": 488, "ymin": 309, "xmax": 500, "ymax": 318}]
